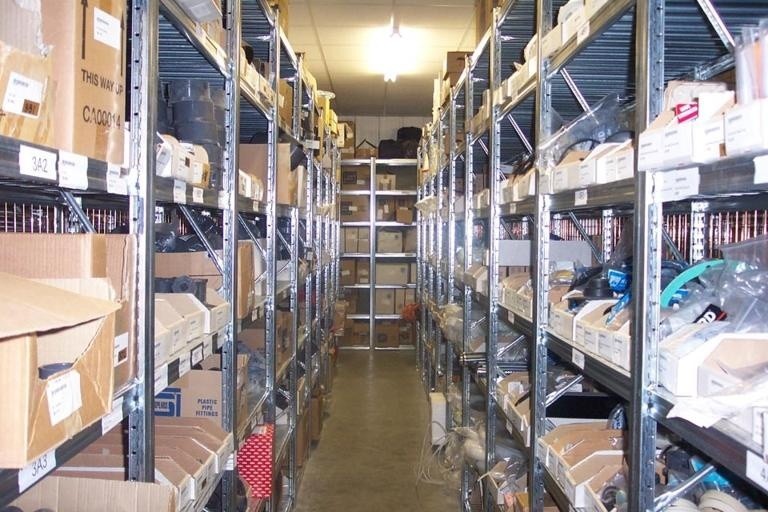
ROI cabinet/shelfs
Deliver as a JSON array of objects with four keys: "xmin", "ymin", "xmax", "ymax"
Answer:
[
  {"xmin": 412, "ymin": 0, "xmax": 768, "ymax": 512},
  {"xmin": 336, "ymin": 136, "xmax": 424, "ymax": 352},
  {"xmin": 1, "ymin": 1, "xmax": 345, "ymax": 511}
]
